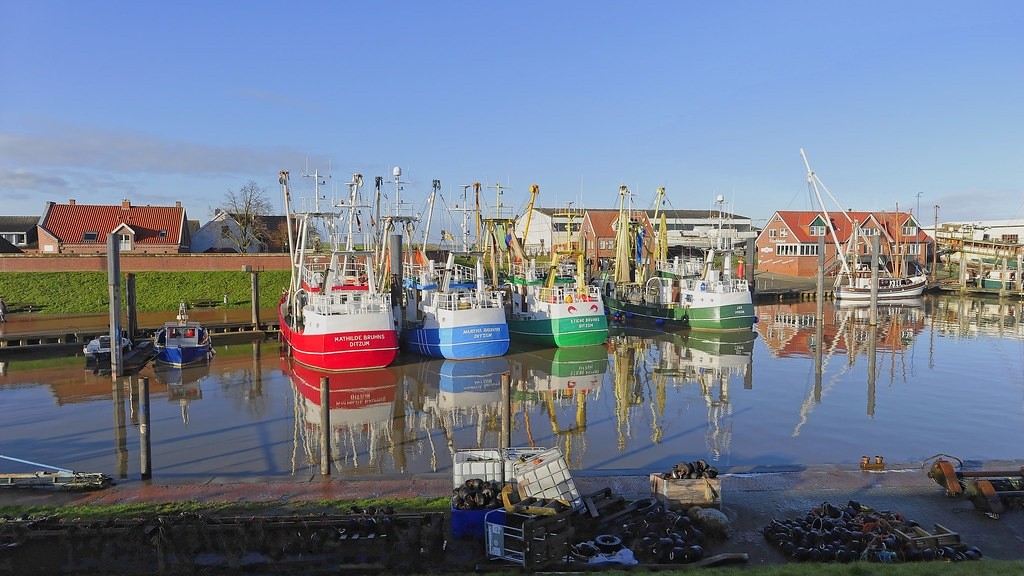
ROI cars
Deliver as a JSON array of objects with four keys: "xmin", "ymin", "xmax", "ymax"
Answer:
[{"xmin": 564, "ymin": 261, "xmax": 577, "ymax": 270}]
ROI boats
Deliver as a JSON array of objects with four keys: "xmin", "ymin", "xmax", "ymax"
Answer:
[
  {"xmin": 82, "ymin": 335, "xmax": 132, "ymax": 363},
  {"xmin": 154, "ymin": 361, "xmax": 210, "ymax": 425},
  {"xmin": 972, "ymin": 267, "xmax": 1024, "ymax": 289},
  {"xmin": 278, "ymin": 155, "xmax": 758, "ymax": 371},
  {"xmin": 279, "ymin": 322, "xmax": 759, "ymax": 478},
  {"xmin": 152, "ymin": 300, "xmax": 212, "ymax": 366}
]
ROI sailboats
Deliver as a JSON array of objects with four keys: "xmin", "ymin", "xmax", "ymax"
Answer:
[
  {"xmin": 791, "ymin": 295, "xmax": 923, "ymax": 438},
  {"xmin": 798, "ymin": 146, "xmax": 928, "ymax": 300}
]
